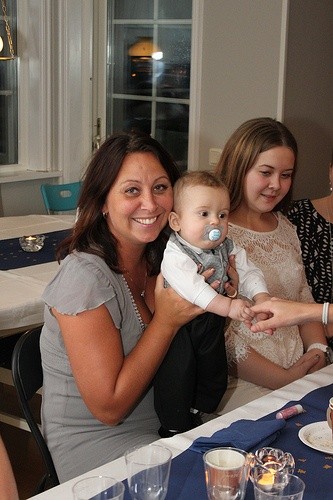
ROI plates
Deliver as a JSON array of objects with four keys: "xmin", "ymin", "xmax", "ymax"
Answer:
[{"xmin": 298, "ymin": 420, "xmax": 333, "ymax": 455}]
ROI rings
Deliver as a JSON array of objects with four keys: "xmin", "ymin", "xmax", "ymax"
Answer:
[{"xmin": 227, "ymin": 290, "xmax": 238, "ymax": 298}]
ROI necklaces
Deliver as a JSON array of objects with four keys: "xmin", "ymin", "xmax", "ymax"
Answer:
[
  {"xmin": 124, "ymin": 268, "xmax": 147, "ymax": 299},
  {"xmin": 122, "ymin": 275, "xmax": 145, "ymax": 333}
]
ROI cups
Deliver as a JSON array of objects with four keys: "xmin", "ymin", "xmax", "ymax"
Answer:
[
  {"xmin": 326, "ymin": 397, "xmax": 333, "ymax": 429},
  {"xmin": 202, "ymin": 445, "xmax": 306, "ymax": 500},
  {"xmin": 72, "ymin": 475, "xmax": 125, "ymax": 500},
  {"xmin": 123, "ymin": 443, "xmax": 172, "ymax": 500}
]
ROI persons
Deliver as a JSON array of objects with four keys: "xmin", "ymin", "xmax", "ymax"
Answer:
[
  {"xmin": 215, "ymin": 117, "xmax": 329, "ymax": 418},
  {"xmin": 153, "ymin": 171, "xmax": 278, "ymax": 437},
  {"xmin": 41, "ymin": 132, "xmax": 239, "ymax": 485},
  {"xmin": 275, "ymin": 149, "xmax": 333, "ymax": 357},
  {"xmin": 242, "ymin": 295, "xmax": 333, "ymax": 336}
]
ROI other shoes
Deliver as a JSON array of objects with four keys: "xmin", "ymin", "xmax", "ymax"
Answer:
[{"xmin": 158, "ymin": 404, "xmax": 204, "ymax": 437}]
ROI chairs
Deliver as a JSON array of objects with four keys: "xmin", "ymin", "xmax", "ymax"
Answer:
[
  {"xmin": 12, "ymin": 325, "xmax": 60, "ymax": 494},
  {"xmin": 41, "ymin": 182, "xmax": 83, "ymax": 215}
]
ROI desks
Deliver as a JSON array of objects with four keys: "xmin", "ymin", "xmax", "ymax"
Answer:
[
  {"xmin": 0, "ymin": 215, "xmax": 75, "ymax": 338},
  {"xmin": 33, "ymin": 363, "xmax": 333, "ymax": 500}
]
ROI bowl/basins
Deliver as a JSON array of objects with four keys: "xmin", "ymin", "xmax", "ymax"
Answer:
[
  {"xmin": 248, "ymin": 446, "xmax": 296, "ymax": 489},
  {"xmin": 19, "ymin": 233, "xmax": 45, "ymax": 253}
]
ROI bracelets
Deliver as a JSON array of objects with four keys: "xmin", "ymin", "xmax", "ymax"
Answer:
[{"xmin": 321, "ymin": 302, "xmax": 329, "ymax": 325}]
